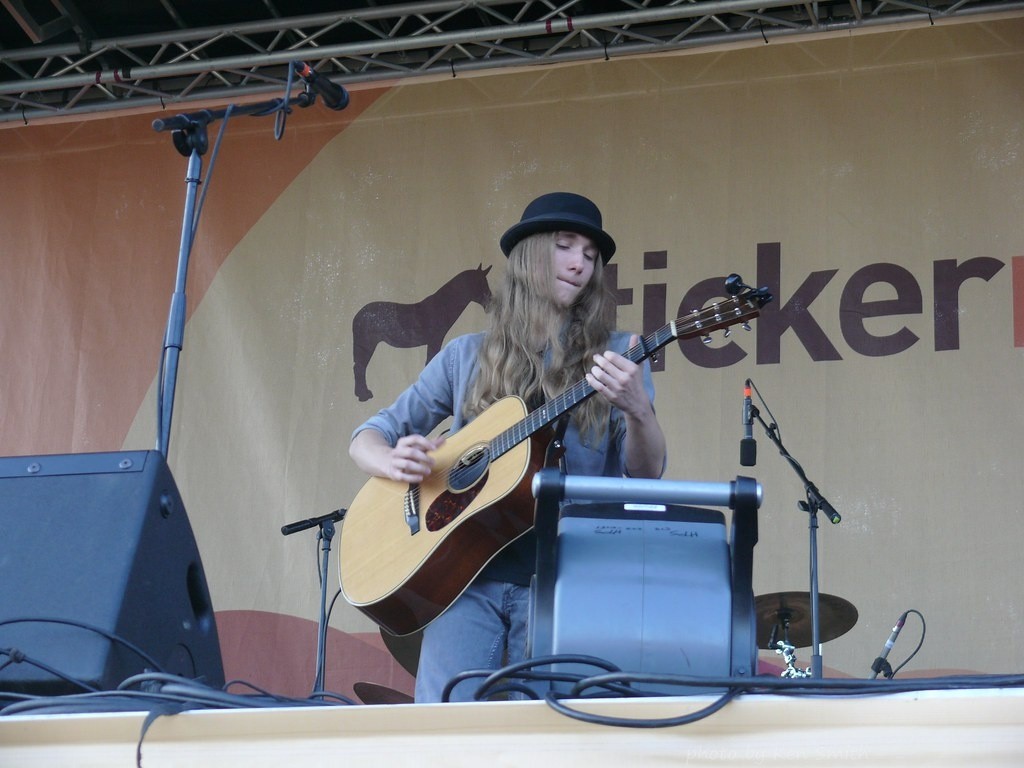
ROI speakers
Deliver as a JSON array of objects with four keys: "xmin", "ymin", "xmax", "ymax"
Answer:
[{"xmin": 0, "ymin": 449, "xmax": 226, "ymax": 700}]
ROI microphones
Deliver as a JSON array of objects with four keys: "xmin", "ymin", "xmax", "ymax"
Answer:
[
  {"xmin": 295, "ymin": 60, "xmax": 350, "ymax": 111},
  {"xmin": 867, "ymin": 611, "xmax": 908, "ymax": 679},
  {"xmin": 739, "ymin": 379, "xmax": 757, "ymax": 467}
]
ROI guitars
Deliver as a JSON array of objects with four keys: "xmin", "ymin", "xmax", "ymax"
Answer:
[{"xmin": 336, "ymin": 272, "xmax": 775, "ymax": 639}]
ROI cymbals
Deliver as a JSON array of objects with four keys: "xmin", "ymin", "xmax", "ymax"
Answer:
[
  {"xmin": 350, "ymin": 681, "xmax": 416, "ymax": 705},
  {"xmin": 752, "ymin": 589, "xmax": 860, "ymax": 651},
  {"xmin": 379, "ymin": 626, "xmax": 424, "ymax": 679}
]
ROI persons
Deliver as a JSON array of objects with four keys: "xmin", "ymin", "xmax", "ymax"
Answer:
[{"xmin": 348, "ymin": 192, "xmax": 667, "ymax": 704}]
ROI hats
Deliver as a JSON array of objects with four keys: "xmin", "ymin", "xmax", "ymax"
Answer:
[{"xmin": 499, "ymin": 192, "xmax": 616, "ymax": 269}]
